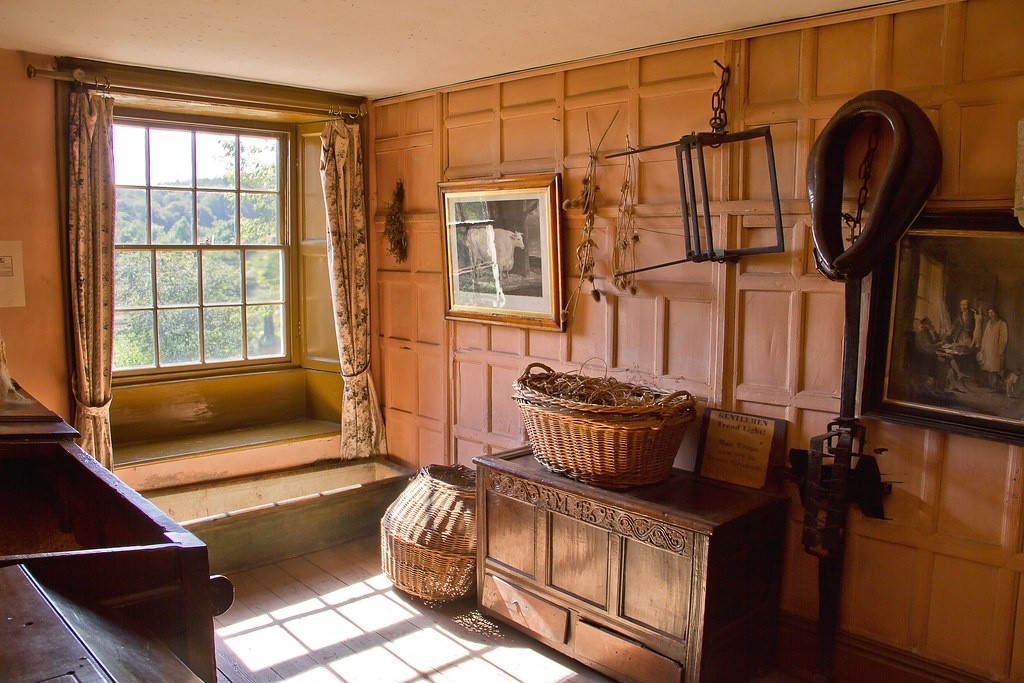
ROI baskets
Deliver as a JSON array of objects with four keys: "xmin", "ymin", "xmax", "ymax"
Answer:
[
  {"xmin": 511, "ymin": 362, "xmax": 697, "ymax": 491},
  {"xmin": 379, "ymin": 464, "xmax": 479, "ymax": 603}
]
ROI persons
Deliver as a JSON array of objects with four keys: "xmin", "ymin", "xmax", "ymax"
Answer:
[
  {"xmin": 976, "ymin": 306, "xmax": 1009, "ymax": 394},
  {"xmin": 914, "ymin": 315, "xmax": 971, "ymax": 390},
  {"xmin": 943, "ymin": 298, "xmax": 985, "ymax": 380}
]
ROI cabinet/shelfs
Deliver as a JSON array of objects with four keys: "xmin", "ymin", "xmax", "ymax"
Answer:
[
  {"xmin": 466, "ymin": 444, "xmax": 792, "ymax": 682},
  {"xmin": 0, "ymin": 372, "xmax": 236, "ymax": 683}
]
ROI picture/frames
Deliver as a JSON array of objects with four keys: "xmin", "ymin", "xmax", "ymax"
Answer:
[
  {"xmin": 434, "ymin": 172, "xmax": 567, "ymax": 334},
  {"xmin": 858, "ymin": 208, "xmax": 1024, "ymax": 449}
]
